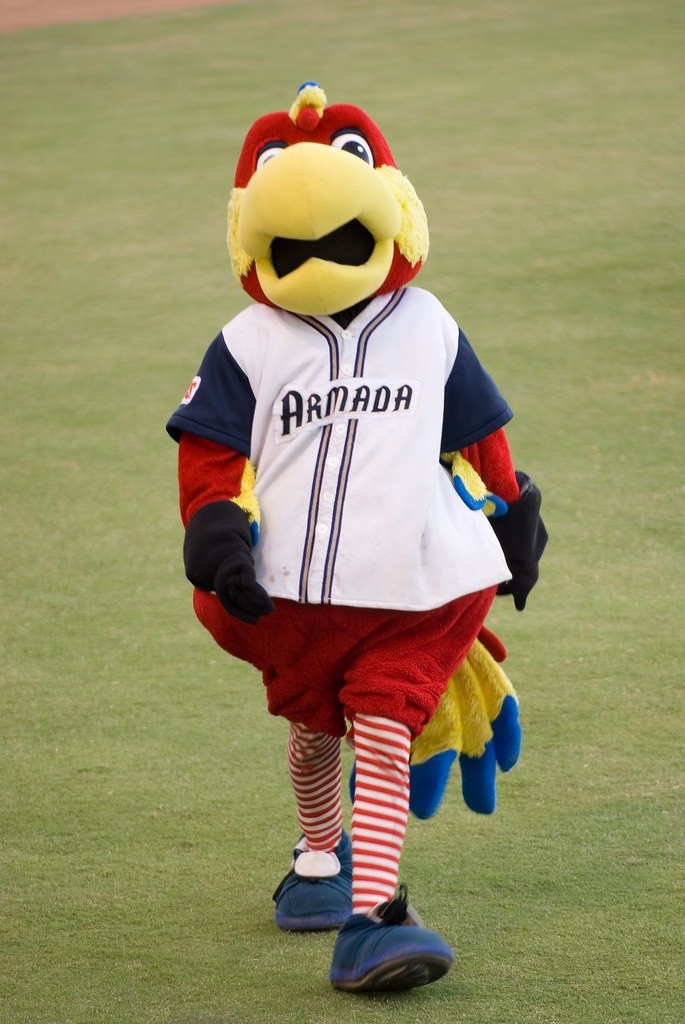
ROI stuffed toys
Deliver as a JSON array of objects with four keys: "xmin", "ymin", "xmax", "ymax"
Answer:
[{"xmin": 166, "ymin": 85, "xmax": 549, "ymax": 992}]
